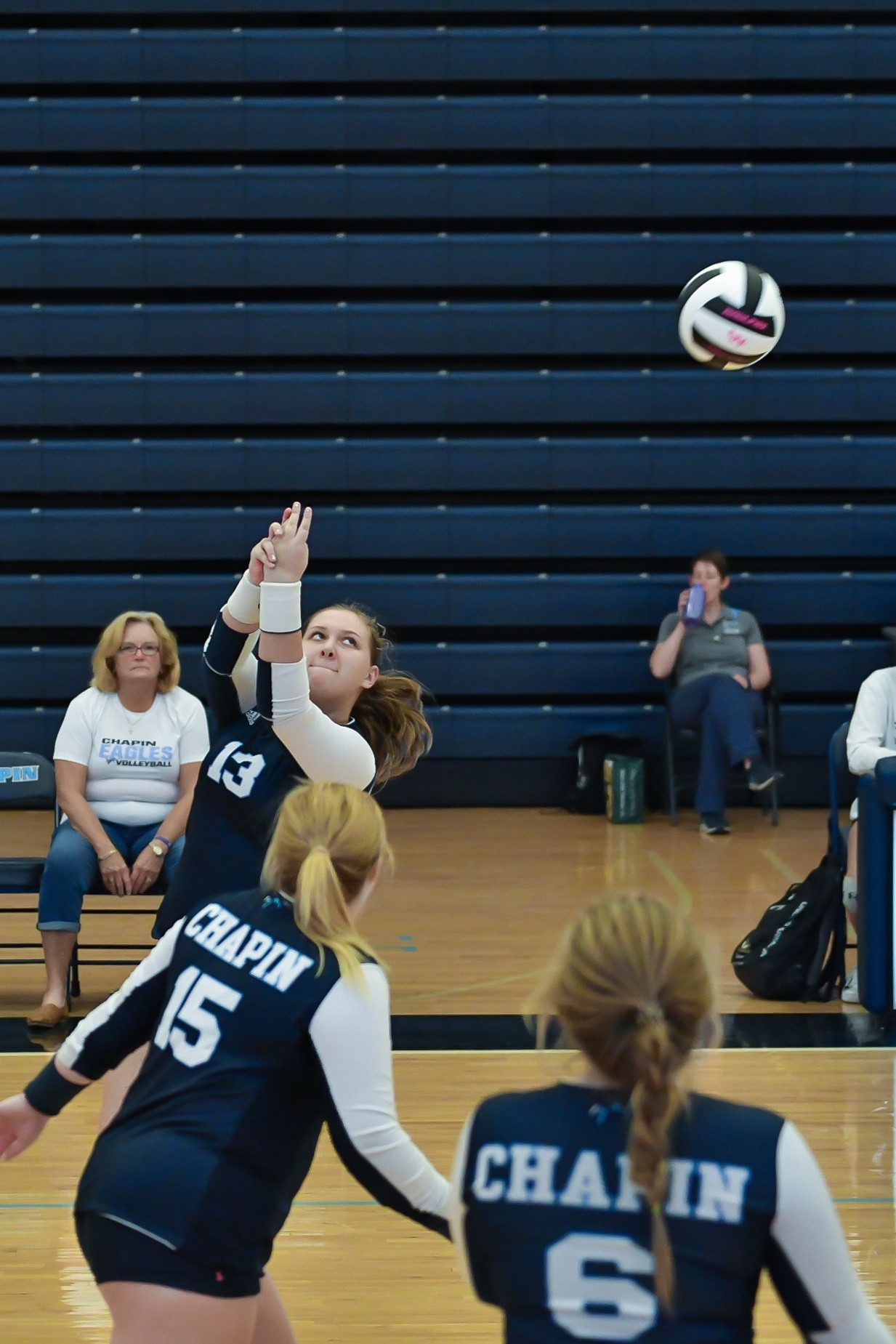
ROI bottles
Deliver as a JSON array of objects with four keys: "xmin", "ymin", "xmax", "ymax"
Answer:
[{"xmin": 686, "ymin": 583, "xmax": 706, "ymax": 626}]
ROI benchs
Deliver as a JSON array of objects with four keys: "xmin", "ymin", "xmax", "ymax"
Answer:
[{"xmin": 1, "ymin": 27, "xmax": 895, "ymax": 809}]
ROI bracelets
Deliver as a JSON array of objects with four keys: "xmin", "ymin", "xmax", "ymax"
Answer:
[
  {"xmin": 743, "ymin": 676, "xmax": 751, "ymax": 690},
  {"xmin": 97, "ymin": 849, "xmax": 117, "ymax": 860},
  {"xmin": 227, "ymin": 568, "xmax": 260, "ymax": 624},
  {"xmin": 259, "ymin": 579, "xmax": 301, "ymax": 633}
]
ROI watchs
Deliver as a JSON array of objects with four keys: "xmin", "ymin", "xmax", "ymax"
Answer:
[
  {"xmin": 148, "ymin": 841, "xmax": 165, "ymax": 859},
  {"xmin": 154, "ymin": 835, "xmax": 171, "ymax": 849}
]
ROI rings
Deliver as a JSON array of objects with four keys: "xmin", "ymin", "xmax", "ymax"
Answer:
[
  {"xmin": 108, "ymin": 878, "xmax": 114, "ymax": 882},
  {"xmin": 147, "ymin": 878, "xmax": 151, "ymax": 882}
]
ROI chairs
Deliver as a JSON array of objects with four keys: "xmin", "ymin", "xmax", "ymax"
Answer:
[
  {"xmin": 0, "ymin": 749, "xmax": 72, "ymax": 1012},
  {"xmin": 73, "ymin": 869, "xmax": 168, "ymax": 996},
  {"xmin": 663, "ymin": 676, "xmax": 780, "ymax": 828},
  {"xmin": 828, "ymin": 721, "xmax": 857, "ymax": 998}
]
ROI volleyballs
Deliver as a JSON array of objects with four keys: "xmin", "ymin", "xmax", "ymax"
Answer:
[{"xmin": 676, "ymin": 260, "xmax": 786, "ymax": 370}]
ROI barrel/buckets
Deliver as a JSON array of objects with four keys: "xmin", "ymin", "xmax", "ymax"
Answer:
[{"xmin": 603, "ymin": 754, "xmax": 646, "ymax": 824}]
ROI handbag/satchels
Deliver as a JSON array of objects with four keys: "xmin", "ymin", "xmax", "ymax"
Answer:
[
  {"xmin": 572, "ymin": 736, "xmax": 645, "ymax": 813},
  {"xmin": 731, "ymin": 845, "xmax": 847, "ymax": 1004}
]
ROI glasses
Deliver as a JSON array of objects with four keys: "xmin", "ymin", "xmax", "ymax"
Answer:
[
  {"xmin": 120, "ymin": 643, "xmax": 159, "ymax": 656},
  {"xmin": 692, "ymin": 573, "xmax": 720, "ymax": 583}
]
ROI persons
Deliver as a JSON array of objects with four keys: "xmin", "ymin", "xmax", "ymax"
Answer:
[
  {"xmin": 0, "ymin": 781, "xmax": 452, "ymax": 1344},
  {"xmin": 648, "ymin": 550, "xmax": 785, "ymax": 836},
  {"xmin": 840, "ymin": 667, "xmax": 896, "ymax": 1005},
  {"xmin": 448, "ymin": 891, "xmax": 892, "ymax": 1344},
  {"xmin": 26, "ymin": 612, "xmax": 211, "ymax": 1027},
  {"xmin": 95, "ymin": 502, "xmax": 433, "ymax": 1131}
]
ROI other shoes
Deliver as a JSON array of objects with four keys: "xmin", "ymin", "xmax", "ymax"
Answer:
[
  {"xmin": 27, "ymin": 999, "xmax": 68, "ymax": 1027},
  {"xmin": 841, "ymin": 966, "xmax": 859, "ymax": 1001},
  {"xmin": 701, "ymin": 813, "xmax": 732, "ymax": 834},
  {"xmin": 747, "ymin": 763, "xmax": 780, "ymax": 789}
]
